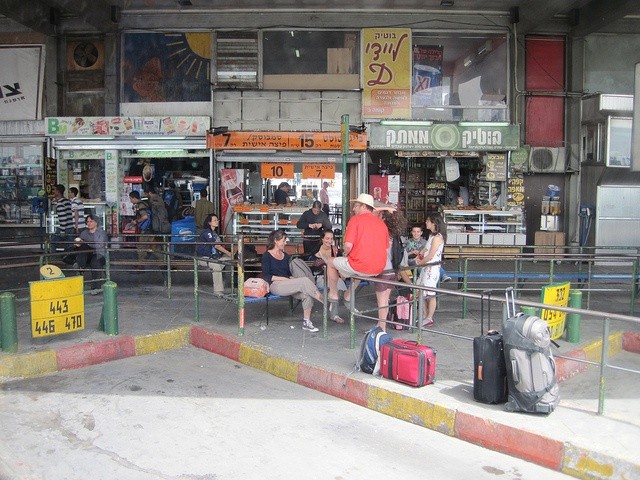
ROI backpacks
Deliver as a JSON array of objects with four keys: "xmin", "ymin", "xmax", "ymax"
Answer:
[
  {"xmin": 356, "ymin": 326, "xmax": 390, "ymax": 376},
  {"xmin": 136, "ymin": 201, "xmax": 171, "ymax": 234}
]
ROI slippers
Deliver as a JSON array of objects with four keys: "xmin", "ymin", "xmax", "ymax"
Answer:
[
  {"xmin": 320, "ymin": 294, "xmax": 338, "ymax": 304},
  {"xmin": 329, "ymin": 313, "xmax": 345, "ymax": 324},
  {"xmin": 338, "ymin": 290, "xmax": 350, "ymax": 303}
]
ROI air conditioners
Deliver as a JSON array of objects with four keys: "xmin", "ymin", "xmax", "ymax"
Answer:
[{"xmin": 528, "ymin": 147, "xmax": 567, "ymax": 174}]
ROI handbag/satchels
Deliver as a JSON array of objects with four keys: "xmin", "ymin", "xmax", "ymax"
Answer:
[
  {"xmin": 391, "ymin": 235, "xmax": 407, "ymax": 270},
  {"xmin": 289, "ymin": 257, "xmax": 316, "ymax": 285},
  {"xmin": 63, "ymin": 244, "xmax": 79, "ymax": 265},
  {"xmin": 307, "ymin": 253, "xmax": 328, "ymax": 275},
  {"xmin": 243, "ymin": 277, "xmax": 270, "ymax": 298}
]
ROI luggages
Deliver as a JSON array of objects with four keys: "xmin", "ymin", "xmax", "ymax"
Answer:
[
  {"xmin": 474, "ymin": 289, "xmax": 508, "ymax": 404},
  {"xmin": 379, "ymin": 339, "xmax": 438, "ymax": 388},
  {"xmin": 233, "ymin": 226, "xmax": 256, "ymax": 288},
  {"xmin": 387, "ymin": 287, "xmax": 418, "ymax": 330},
  {"xmin": 502, "ymin": 286, "xmax": 561, "ymax": 415}
]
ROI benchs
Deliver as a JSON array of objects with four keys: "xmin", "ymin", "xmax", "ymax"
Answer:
[{"xmin": 243, "ymin": 279, "xmax": 369, "ymax": 314}]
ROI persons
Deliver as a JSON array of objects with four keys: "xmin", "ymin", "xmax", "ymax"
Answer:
[
  {"xmin": 296, "ymin": 200, "xmax": 332, "ymax": 256},
  {"xmin": 364, "ymin": 207, "xmax": 406, "ymax": 336},
  {"xmin": 406, "ymin": 224, "xmax": 451, "ymax": 282},
  {"xmin": 195, "ymin": 188, "xmax": 214, "ymax": 256},
  {"xmin": 321, "ymin": 193, "xmax": 390, "ymax": 303},
  {"xmin": 274, "ymin": 182, "xmax": 292, "ymax": 231},
  {"xmin": 309, "ymin": 229, "xmax": 362, "ymax": 324},
  {"xmin": 68, "ymin": 187, "xmax": 86, "ymax": 237},
  {"xmin": 320, "ymin": 182, "xmax": 330, "ymax": 219},
  {"xmin": 72, "ymin": 215, "xmax": 107, "ymax": 295},
  {"xmin": 194, "ymin": 214, "xmax": 232, "ymax": 296},
  {"xmin": 129, "ymin": 191, "xmax": 175, "ymax": 273},
  {"xmin": 261, "ymin": 230, "xmax": 332, "ymax": 333},
  {"xmin": 47, "ymin": 184, "xmax": 75, "ymax": 261},
  {"xmin": 415, "ymin": 213, "xmax": 445, "ymax": 327}
]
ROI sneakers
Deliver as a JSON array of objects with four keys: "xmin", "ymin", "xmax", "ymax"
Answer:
[
  {"xmin": 415, "ymin": 319, "xmax": 433, "ymax": 326},
  {"xmin": 442, "ymin": 274, "xmax": 453, "ymax": 282},
  {"xmin": 302, "ymin": 319, "xmax": 319, "ymax": 333}
]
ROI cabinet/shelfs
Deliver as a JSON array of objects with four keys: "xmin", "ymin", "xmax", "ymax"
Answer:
[
  {"xmin": 427, "ymin": 176, "xmax": 445, "ymax": 212},
  {"xmin": 443, "ymin": 210, "xmax": 527, "ymax": 247},
  {"xmin": 399, "ymin": 169, "xmax": 427, "ymax": 224},
  {"xmin": 232, "ymin": 205, "xmax": 304, "ymax": 237}
]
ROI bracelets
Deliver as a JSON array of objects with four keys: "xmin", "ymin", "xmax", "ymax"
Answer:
[{"xmin": 136, "ymin": 219, "xmax": 139, "ymax": 224}]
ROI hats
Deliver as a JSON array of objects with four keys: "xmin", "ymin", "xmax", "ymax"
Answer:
[{"xmin": 349, "ymin": 193, "xmax": 377, "ymax": 211}]
